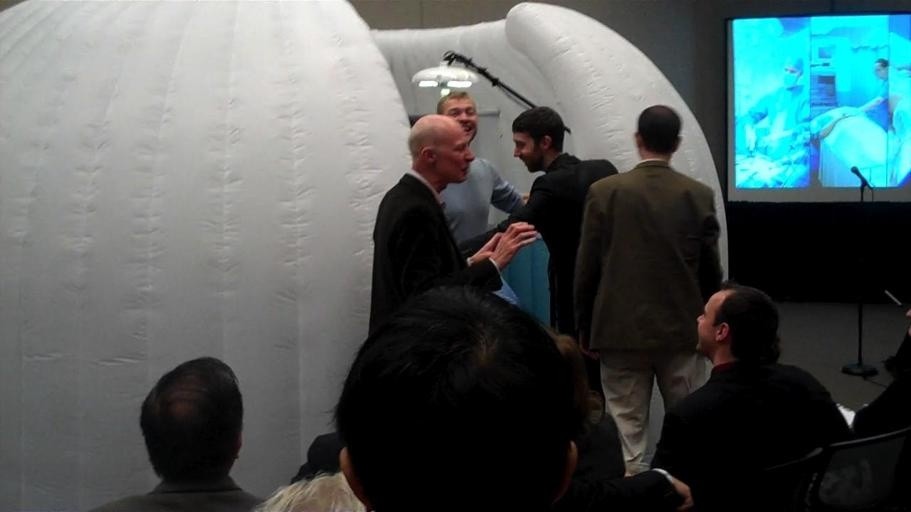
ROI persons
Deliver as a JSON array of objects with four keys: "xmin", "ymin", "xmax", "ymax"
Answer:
[
  {"xmin": 742, "ymin": 57, "xmax": 810, "ymax": 165},
  {"xmin": 87, "ymin": 355, "xmax": 266, "ymax": 512},
  {"xmin": 809, "ymin": 112, "xmax": 850, "ymax": 150},
  {"xmin": 573, "ymin": 104, "xmax": 725, "ymax": 477},
  {"xmin": 457, "ymin": 106, "xmax": 619, "ymax": 415},
  {"xmin": 648, "ymin": 277, "xmax": 853, "ymax": 512},
  {"xmin": 366, "ymin": 112, "xmax": 538, "ymax": 335},
  {"xmin": 859, "ymin": 57, "xmax": 888, "ymax": 113},
  {"xmin": 435, "ymin": 90, "xmax": 526, "ymax": 243}
]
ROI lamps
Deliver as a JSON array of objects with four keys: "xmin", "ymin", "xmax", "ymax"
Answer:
[{"xmin": 411, "ymin": 49, "xmax": 572, "ymax": 136}]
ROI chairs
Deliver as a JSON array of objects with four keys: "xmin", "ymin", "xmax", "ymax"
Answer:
[
  {"xmin": 820, "ymin": 426, "xmax": 911, "ymax": 512},
  {"xmin": 728, "ymin": 445, "xmax": 825, "ymax": 510}
]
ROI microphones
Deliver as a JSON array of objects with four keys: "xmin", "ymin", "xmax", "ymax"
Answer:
[{"xmin": 850, "ymin": 165, "xmax": 873, "ymax": 192}]
